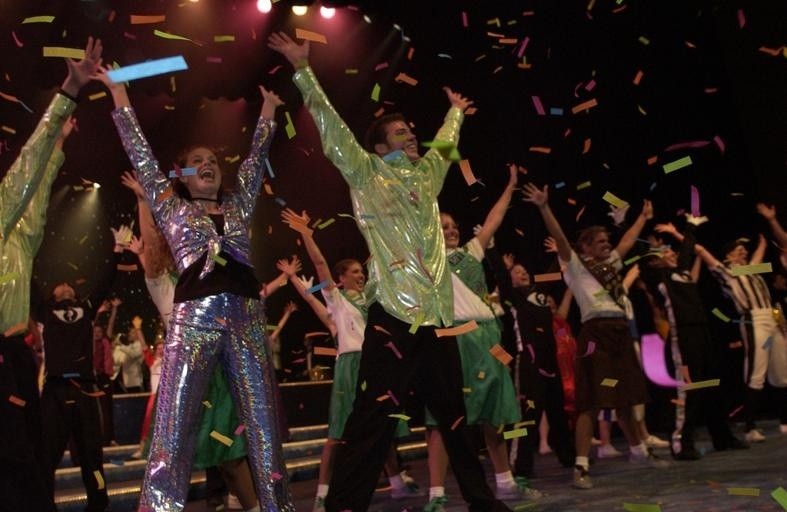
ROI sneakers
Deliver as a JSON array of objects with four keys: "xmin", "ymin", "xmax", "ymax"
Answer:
[
  {"xmin": 645, "ymin": 435, "xmax": 670, "ymax": 447},
  {"xmin": 495, "ymin": 477, "xmax": 541, "ymax": 500},
  {"xmin": 424, "ymin": 494, "xmax": 450, "ymax": 512},
  {"xmin": 780, "ymin": 424, "xmax": 787, "ymax": 433},
  {"xmin": 313, "ymin": 496, "xmax": 326, "ymax": 512},
  {"xmin": 745, "ymin": 428, "xmax": 766, "ymax": 442},
  {"xmin": 390, "ymin": 481, "xmax": 426, "ymax": 499},
  {"xmin": 400, "ymin": 470, "xmax": 414, "ymax": 483},
  {"xmin": 598, "ymin": 444, "xmax": 622, "ymax": 457},
  {"xmin": 574, "ymin": 465, "xmax": 593, "ymax": 489},
  {"xmin": 629, "ymin": 447, "xmax": 668, "ymax": 469}
]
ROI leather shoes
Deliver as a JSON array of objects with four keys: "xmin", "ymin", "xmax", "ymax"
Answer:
[
  {"xmin": 671, "ymin": 450, "xmax": 701, "ymax": 460},
  {"xmin": 712, "ymin": 434, "xmax": 750, "ymax": 450}
]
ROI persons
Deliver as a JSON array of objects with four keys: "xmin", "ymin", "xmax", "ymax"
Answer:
[
  {"xmin": 0, "ymin": 34, "xmax": 105, "ymax": 512},
  {"xmin": 268, "ymin": 30, "xmax": 510, "ymax": 510},
  {"xmin": 0, "ymin": 160, "xmax": 787, "ymax": 511},
  {"xmin": 87, "ymin": 59, "xmax": 296, "ymax": 511}
]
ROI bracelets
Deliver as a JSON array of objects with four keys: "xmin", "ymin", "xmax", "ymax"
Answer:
[{"xmin": 294, "ymin": 58, "xmax": 309, "ymax": 70}]
